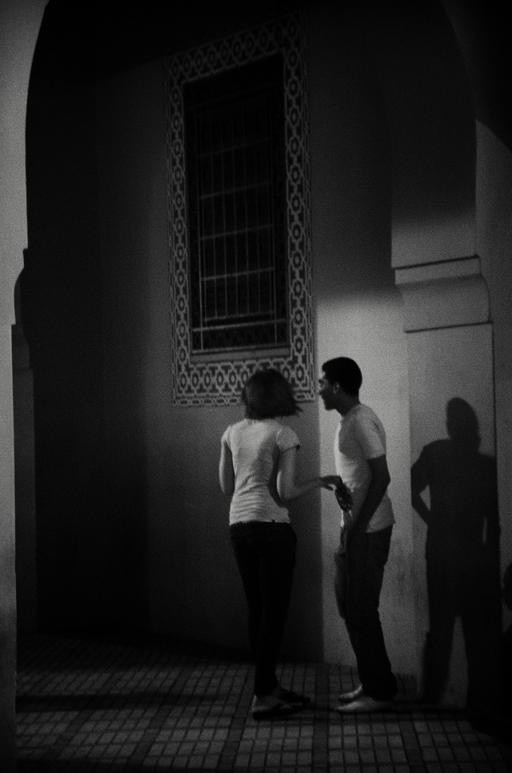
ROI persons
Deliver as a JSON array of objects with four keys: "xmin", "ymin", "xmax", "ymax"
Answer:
[
  {"xmin": 217, "ymin": 367, "xmax": 337, "ymax": 719},
  {"xmin": 320, "ymin": 355, "xmax": 400, "ymax": 713}
]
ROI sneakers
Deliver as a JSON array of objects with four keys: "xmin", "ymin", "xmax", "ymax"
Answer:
[{"xmin": 247, "ymin": 680, "xmax": 397, "ymax": 719}]
